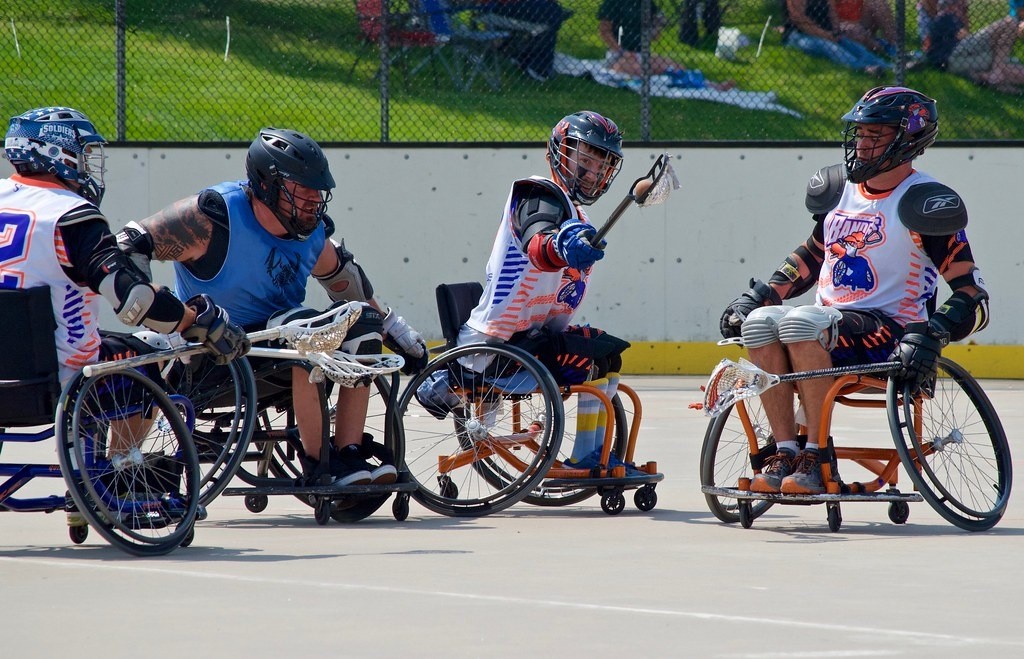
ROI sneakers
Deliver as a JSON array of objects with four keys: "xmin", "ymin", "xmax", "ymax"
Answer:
[
  {"xmin": 780, "ymin": 438, "xmax": 840, "ymax": 495},
  {"xmin": 750, "ymin": 435, "xmax": 807, "ymax": 493}
]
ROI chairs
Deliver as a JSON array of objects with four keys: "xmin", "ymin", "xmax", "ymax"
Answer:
[{"xmin": 347, "ymin": 0, "xmax": 509, "ymax": 95}]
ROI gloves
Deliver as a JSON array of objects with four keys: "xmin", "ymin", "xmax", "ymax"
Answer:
[
  {"xmin": 720, "ymin": 278, "xmax": 782, "ymax": 350},
  {"xmin": 552, "ymin": 219, "xmax": 607, "ymax": 271},
  {"xmin": 188, "ymin": 293, "xmax": 251, "ymax": 366},
  {"xmin": 383, "ymin": 307, "xmax": 429, "ymax": 377},
  {"xmin": 887, "ymin": 328, "xmax": 950, "ymax": 398}
]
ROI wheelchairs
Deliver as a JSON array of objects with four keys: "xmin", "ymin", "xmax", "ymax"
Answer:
[
  {"xmin": 57, "ymin": 302, "xmax": 404, "ymax": 550},
  {"xmin": 390, "ymin": 281, "xmax": 662, "ymax": 518},
  {"xmin": 0, "ymin": 286, "xmax": 200, "ymax": 545},
  {"xmin": 699, "ymin": 307, "xmax": 1010, "ymax": 531}
]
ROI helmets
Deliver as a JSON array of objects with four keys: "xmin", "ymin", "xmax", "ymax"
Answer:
[
  {"xmin": 246, "ymin": 127, "xmax": 336, "ymax": 241},
  {"xmin": 842, "ymin": 87, "xmax": 939, "ymax": 183},
  {"xmin": 546, "ymin": 111, "xmax": 624, "ymax": 206},
  {"xmin": 5, "ymin": 107, "xmax": 108, "ymax": 206}
]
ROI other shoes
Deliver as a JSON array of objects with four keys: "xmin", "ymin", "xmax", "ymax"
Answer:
[
  {"xmin": 907, "ymin": 60, "xmax": 926, "ymax": 76},
  {"xmin": 338, "ymin": 456, "xmax": 397, "ymax": 484},
  {"xmin": 510, "ymin": 57, "xmax": 526, "ymax": 72},
  {"xmin": 873, "ymin": 64, "xmax": 887, "ymax": 81},
  {"xmin": 600, "ymin": 449, "xmax": 649, "ymax": 477},
  {"xmin": 527, "ymin": 66, "xmax": 550, "ymax": 82},
  {"xmin": 564, "ymin": 452, "xmax": 601, "ymax": 476},
  {"xmin": 301, "ymin": 466, "xmax": 373, "ymax": 488}
]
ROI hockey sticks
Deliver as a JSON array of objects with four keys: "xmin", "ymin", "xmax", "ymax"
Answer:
[
  {"xmin": 703, "ymin": 357, "xmax": 900, "ymax": 418},
  {"xmin": 589, "ymin": 153, "xmax": 681, "ymax": 247},
  {"xmin": 83, "ymin": 299, "xmax": 406, "ymax": 386}
]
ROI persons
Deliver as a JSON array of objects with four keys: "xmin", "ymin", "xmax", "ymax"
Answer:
[
  {"xmin": 1, "ymin": 106, "xmax": 251, "ymax": 529},
  {"xmin": 680, "ymin": 0, "xmax": 719, "ymax": 52},
  {"xmin": 486, "ymin": 0, "xmax": 563, "ymax": 81},
  {"xmin": 918, "ymin": 0, "xmax": 1024, "ymax": 97},
  {"xmin": 597, "ymin": 0, "xmax": 685, "ymax": 76},
  {"xmin": 114, "ymin": 127, "xmax": 428, "ymax": 486},
  {"xmin": 457, "ymin": 110, "xmax": 649, "ymax": 478},
  {"xmin": 782, "ymin": 0, "xmax": 917, "ymax": 78},
  {"xmin": 719, "ymin": 86, "xmax": 989, "ymax": 493}
]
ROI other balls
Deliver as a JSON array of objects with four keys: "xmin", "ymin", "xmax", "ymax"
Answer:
[{"xmin": 635, "ymin": 179, "xmax": 652, "ymax": 197}]
